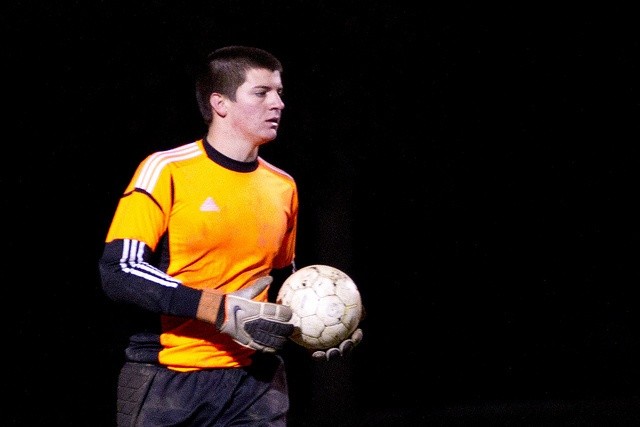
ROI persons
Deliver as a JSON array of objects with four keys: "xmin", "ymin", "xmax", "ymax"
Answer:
[{"xmin": 98, "ymin": 45, "xmax": 364, "ymax": 426}]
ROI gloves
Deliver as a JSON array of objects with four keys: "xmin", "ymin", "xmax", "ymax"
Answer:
[
  {"xmin": 214, "ymin": 276, "xmax": 295, "ymax": 354},
  {"xmin": 312, "ymin": 306, "xmax": 367, "ymax": 363}
]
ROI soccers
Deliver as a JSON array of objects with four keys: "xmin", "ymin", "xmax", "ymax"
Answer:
[{"xmin": 276, "ymin": 264, "xmax": 361, "ymax": 350}]
